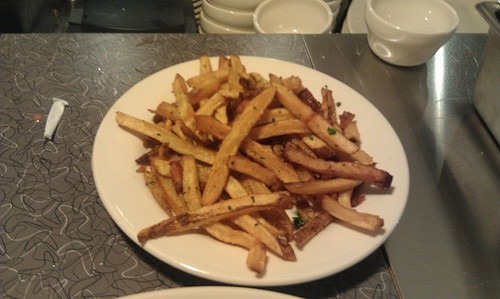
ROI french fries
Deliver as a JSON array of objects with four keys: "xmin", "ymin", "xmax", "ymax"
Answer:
[{"xmin": 116, "ymin": 53, "xmax": 394, "ymax": 275}]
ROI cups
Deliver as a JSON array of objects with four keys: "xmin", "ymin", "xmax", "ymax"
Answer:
[
  {"xmin": 198, "ymin": 1, "xmax": 342, "ymax": 35},
  {"xmin": 363, "ymin": 1, "xmax": 459, "ymax": 67}
]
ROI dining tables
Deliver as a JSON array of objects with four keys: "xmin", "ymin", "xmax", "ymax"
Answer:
[
  {"xmin": 1, "ymin": 31, "xmax": 403, "ymax": 299},
  {"xmin": 302, "ymin": 30, "xmax": 500, "ymax": 299}
]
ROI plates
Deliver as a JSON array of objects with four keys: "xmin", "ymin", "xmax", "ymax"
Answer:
[
  {"xmin": 343, "ymin": 0, "xmax": 498, "ymax": 33},
  {"xmin": 92, "ymin": 55, "xmax": 410, "ymax": 286}
]
